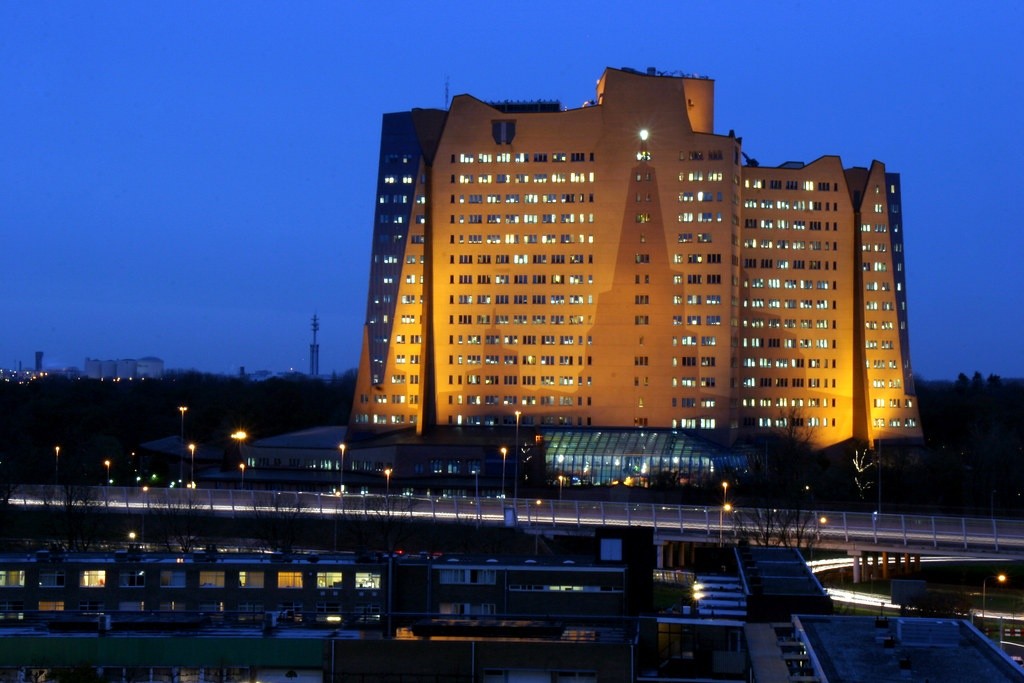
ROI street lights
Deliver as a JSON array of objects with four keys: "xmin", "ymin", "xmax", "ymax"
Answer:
[
  {"xmin": 239, "ymin": 463, "xmax": 245, "ymax": 489},
  {"xmin": 982, "ymin": 574, "xmax": 1006, "ymax": 627},
  {"xmin": 721, "ymin": 482, "xmax": 729, "ymax": 503},
  {"xmin": 104, "ymin": 460, "xmax": 111, "ymax": 506},
  {"xmin": 178, "ymin": 407, "xmax": 188, "ymax": 488},
  {"xmin": 339, "ymin": 444, "xmax": 346, "ymax": 493},
  {"xmin": 535, "ymin": 499, "xmax": 541, "ymax": 557},
  {"xmin": 514, "ymin": 410, "xmax": 520, "ymax": 508},
  {"xmin": 500, "ymin": 447, "xmax": 507, "ymax": 494},
  {"xmin": 384, "ymin": 469, "xmax": 391, "ymax": 507},
  {"xmin": 558, "ymin": 476, "xmax": 563, "ymax": 506},
  {"xmin": 720, "ymin": 503, "xmax": 731, "ymax": 548},
  {"xmin": 55, "ymin": 446, "xmax": 60, "ymax": 484},
  {"xmin": 141, "ymin": 486, "xmax": 149, "ymax": 544},
  {"xmin": 188, "ymin": 443, "xmax": 196, "ymax": 488}
]
